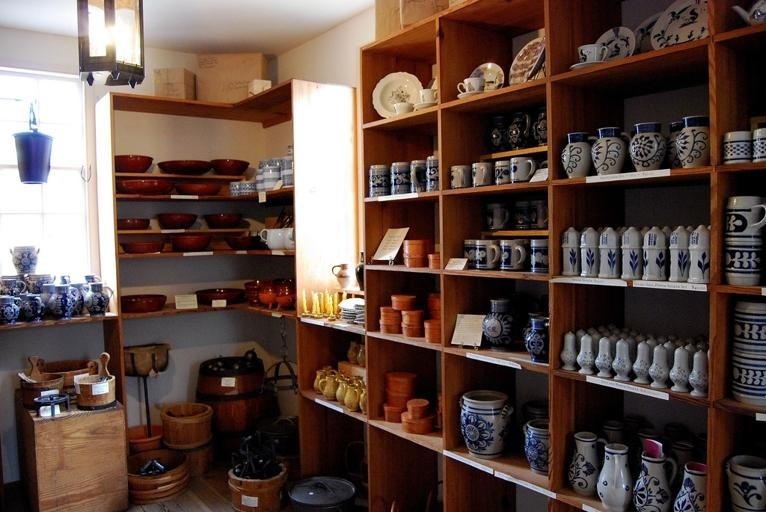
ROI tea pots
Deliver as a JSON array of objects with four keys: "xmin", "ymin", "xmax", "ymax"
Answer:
[
  {"xmin": 596, "ymin": 443, "xmax": 633, "ymax": 512},
  {"xmin": 313, "ymin": 340, "xmax": 366, "ymax": 416},
  {"xmin": 633, "ymin": 451, "xmax": 677, "ymax": 512},
  {"xmin": 567, "ymin": 432, "xmax": 608, "ymax": 496},
  {"xmin": 730, "ymin": 0, "xmax": 766, "ymax": 26},
  {"xmin": 673, "ymin": 461, "xmax": 708, "ymax": 512},
  {"xmin": 80, "ymin": 282, "xmax": 113, "ymax": 317}
]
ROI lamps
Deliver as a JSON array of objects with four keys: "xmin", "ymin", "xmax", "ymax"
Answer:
[{"xmin": 77, "ymin": 1, "xmax": 145, "ymax": 88}]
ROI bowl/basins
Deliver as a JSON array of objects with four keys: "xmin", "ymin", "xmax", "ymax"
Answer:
[{"xmin": 394, "ymin": 103, "xmax": 414, "ymax": 113}]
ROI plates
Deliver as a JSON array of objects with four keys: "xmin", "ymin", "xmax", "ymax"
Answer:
[
  {"xmin": 204, "ymin": 214, "xmax": 242, "ymax": 228},
  {"xmin": 457, "ymin": 91, "xmax": 482, "ymax": 98},
  {"xmin": 527, "ymin": 44, "xmax": 546, "ymax": 78},
  {"xmin": 157, "ymin": 159, "xmax": 212, "ymax": 175},
  {"xmin": 595, "ymin": 27, "xmax": 636, "ymax": 57},
  {"xmin": 339, "ymin": 298, "xmax": 365, "ymax": 321},
  {"xmin": 509, "ymin": 38, "xmax": 546, "ymax": 85},
  {"xmin": 121, "ymin": 295, "xmax": 167, "ymax": 312},
  {"xmin": 117, "ymin": 219, "xmax": 149, "ymax": 230},
  {"xmin": 213, "ymin": 159, "xmax": 249, "ymax": 174},
  {"xmin": 115, "ymin": 155, "xmax": 152, "ymax": 172},
  {"xmin": 158, "ymin": 213, "xmax": 198, "ymax": 229},
  {"xmin": 196, "ymin": 288, "xmax": 244, "ymax": 304},
  {"xmin": 173, "ymin": 236, "xmax": 212, "ymax": 251},
  {"xmin": 651, "ymin": 0, "xmax": 709, "ymax": 50},
  {"xmin": 120, "ymin": 241, "xmax": 164, "ymax": 253},
  {"xmin": 174, "ymin": 183, "xmax": 221, "ymax": 195},
  {"xmin": 469, "ymin": 62, "xmax": 505, "ymax": 91},
  {"xmin": 417, "ymin": 101, "xmax": 436, "ymax": 110},
  {"xmin": 569, "ymin": 61, "xmax": 608, "ymax": 70},
  {"xmin": 116, "ymin": 180, "xmax": 175, "ymax": 193},
  {"xmin": 354, "ymin": 303, "xmax": 365, "ymax": 324},
  {"xmin": 224, "ymin": 236, "xmax": 260, "ymax": 248},
  {"xmin": 372, "ymin": 71, "xmax": 423, "ymax": 118},
  {"xmin": 634, "ymin": 12, "xmax": 665, "ymax": 52}
]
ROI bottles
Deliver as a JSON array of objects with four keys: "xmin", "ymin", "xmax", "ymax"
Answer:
[{"xmin": 354, "ymin": 251, "xmax": 364, "ymax": 292}]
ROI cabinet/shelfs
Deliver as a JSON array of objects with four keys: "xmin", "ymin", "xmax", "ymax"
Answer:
[
  {"xmin": 14, "ymin": 388, "xmax": 129, "ymax": 512},
  {"xmin": 359, "ymin": 0, "xmax": 766, "ymax": 512},
  {"xmin": 96, "ymin": 79, "xmax": 357, "ymax": 512},
  {"xmin": 299, "ymin": 316, "xmax": 366, "ymax": 424}
]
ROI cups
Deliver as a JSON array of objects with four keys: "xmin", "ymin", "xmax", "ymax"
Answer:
[
  {"xmin": 457, "ymin": 77, "xmax": 485, "ymax": 93},
  {"xmin": 753, "ymin": 128, "xmax": 766, "ymax": 163},
  {"xmin": 530, "ymin": 239, "xmax": 548, "ymax": 269},
  {"xmin": 726, "ymin": 195, "xmax": 766, "ymax": 241},
  {"xmin": 451, "ymin": 166, "xmax": 471, "ymax": 188},
  {"xmin": 464, "ymin": 239, "xmax": 475, "ymax": 266},
  {"xmin": 532, "ymin": 201, "xmax": 547, "ymax": 228},
  {"xmin": 472, "ymin": 162, "xmax": 493, "ymax": 186},
  {"xmin": 487, "ymin": 203, "xmax": 509, "ymax": 229},
  {"xmin": 476, "ymin": 240, "xmax": 500, "ymax": 269},
  {"xmin": 419, "ymin": 89, "xmax": 438, "ymax": 104},
  {"xmin": 500, "ymin": 240, "xmax": 529, "ymax": 270},
  {"xmin": 255, "ymin": 169, "xmax": 264, "ymax": 192},
  {"xmin": 263, "ymin": 167, "xmax": 281, "ymax": 190},
  {"xmin": 268, "ymin": 158, "xmax": 281, "ymax": 171},
  {"xmin": 261, "ymin": 229, "xmax": 283, "ymax": 249},
  {"xmin": 513, "ymin": 201, "xmax": 538, "ymax": 229},
  {"xmin": 578, "ymin": 44, "xmax": 609, "ymax": 62},
  {"xmin": 259, "ymin": 160, "xmax": 268, "ymax": 169},
  {"xmin": 510, "ymin": 157, "xmax": 535, "ymax": 182},
  {"xmin": 724, "ymin": 131, "xmax": 752, "ymax": 164},
  {"xmin": 229, "ymin": 182, "xmax": 239, "ymax": 196},
  {"xmin": 239, "ymin": 181, "xmax": 255, "ymax": 195},
  {"xmin": 494, "ymin": 161, "xmax": 511, "ymax": 184},
  {"xmin": 280, "ymin": 156, "xmax": 292, "ymax": 169},
  {"xmin": 283, "ymin": 228, "xmax": 294, "ymax": 250}
]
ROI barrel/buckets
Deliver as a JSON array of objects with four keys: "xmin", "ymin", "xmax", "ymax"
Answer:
[
  {"xmin": 288, "ymin": 476, "xmax": 356, "ymax": 512},
  {"xmin": 228, "ymin": 463, "xmax": 287, "ymax": 512},
  {"xmin": 197, "ymin": 353, "xmax": 278, "ymax": 468}
]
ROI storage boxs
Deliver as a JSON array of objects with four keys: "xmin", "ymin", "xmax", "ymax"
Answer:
[
  {"xmin": 152, "ymin": 67, "xmax": 195, "ymax": 100},
  {"xmin": 196, "ymin": 52, "xmax": 270, "ymax": 104}
]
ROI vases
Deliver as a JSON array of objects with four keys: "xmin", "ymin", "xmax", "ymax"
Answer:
[
  {"xmin": 0, "ymin": 246, "xmax": 114, "ymax": 324},
  {"xmin": 569, "ymin": 432, "xmax": 707, "ymax": 512},
  {"xmin": 561, "ymin": 114, "xmax": 710, "ymax": 179},
  {"xmin": 726, "ymin": 455, "xmax": 766, "ymax": 512},
  {"xmin": 483, "ymin": 299, "xmax": 550, "ymax": 363},
  {"xmin": 459, "ymin": 390, "xmax": 514, "ymax": 459},
  {"xmin": 523, "ymin": 418, "xmax": 549, "ymax": 476}
]
